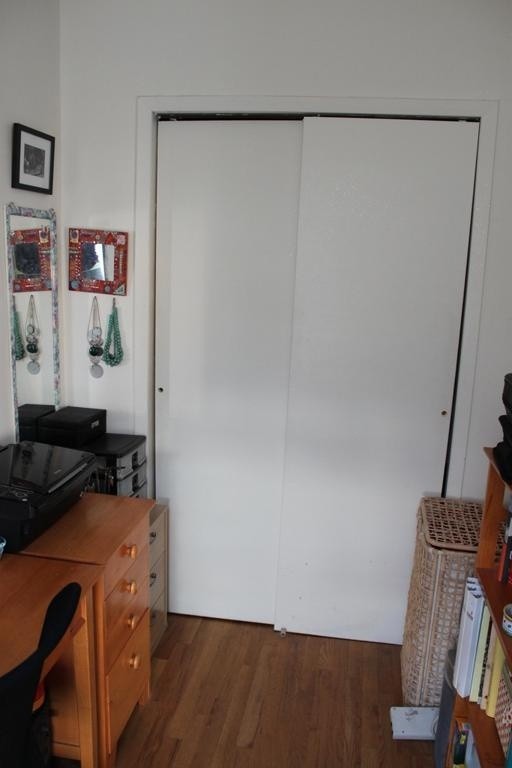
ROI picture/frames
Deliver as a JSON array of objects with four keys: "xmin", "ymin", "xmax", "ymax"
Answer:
[{"xmin": 12, "ymin": 122, "xmax": 56, "ymax": 195}]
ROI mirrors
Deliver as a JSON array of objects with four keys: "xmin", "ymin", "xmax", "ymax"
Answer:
[
  {"xmin": 10, "ymin": 228, "xmax": 52, "ymax": 295},
  {"xmin": 5, "ymin": 205, "xmax": 59, "ymax": 445},
  {"xmin": 68, "ymin": 227, "xmax": 128, "ymax": 296}
]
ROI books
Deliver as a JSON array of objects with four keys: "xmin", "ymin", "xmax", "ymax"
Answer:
[{"xmin": 447, "ymin": 577, "xmax": 505, "ymax": 767}]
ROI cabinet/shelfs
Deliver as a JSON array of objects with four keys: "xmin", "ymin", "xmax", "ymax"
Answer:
[
  {"xmin": 148, "ymin": 504, "xmax": 169, "ymax": 658},
  {"xmin": 433, "ymin": 443, "xmax": 512, "ymax": 768},
  {"xmin": 19, "ymin": 492, "xmax": 157, "ymax": 768}
]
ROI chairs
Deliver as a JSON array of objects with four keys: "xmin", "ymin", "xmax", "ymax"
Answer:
[{"xmin": 0, "ymin": 582, "xmax": 80, "ymax": 768}]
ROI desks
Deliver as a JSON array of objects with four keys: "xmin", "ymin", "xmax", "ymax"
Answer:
[{"xmin": 0, "ymin": 553, "xmax": 106, "ymax": 768}]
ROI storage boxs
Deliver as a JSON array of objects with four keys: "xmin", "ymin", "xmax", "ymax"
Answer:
[
  {"xmin": 19, "ymin": 404, "xmax": 55, "ymax": 441},
  {"xmin": 95, "ymin": 433, "xmax": 148, "ymax": 498},
  {"xmin": 39, "ymin": 407, "xmax": 106, "ymax": 451}
]
